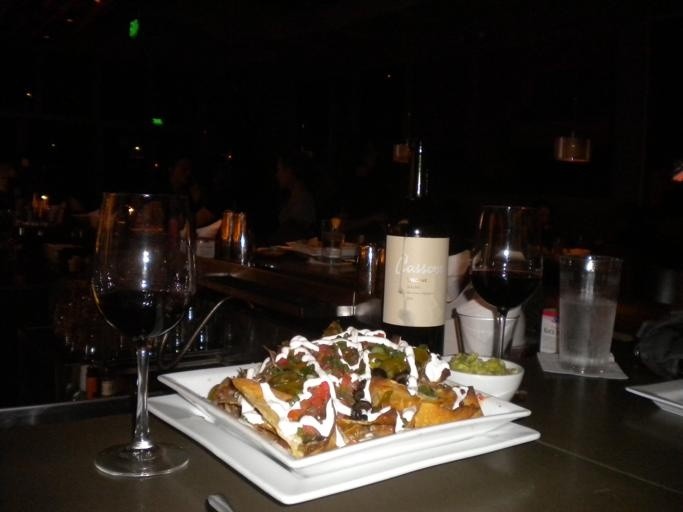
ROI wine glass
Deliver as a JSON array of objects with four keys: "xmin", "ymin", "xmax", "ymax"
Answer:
[
  {"xmin": 93, "ymin": 194, "xmax": 197, "ymax": 479},
  {"xmin": 467, "ymin": 204, "xmax": 542, "ymax": 359}
]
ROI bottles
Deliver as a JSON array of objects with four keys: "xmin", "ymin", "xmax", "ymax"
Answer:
[
  {"xmin": 214, "ymin": 209, "xmax": 249, "ymax": 264},
  {"xmin": 383, "ymin": 131, "xmax": 448, "ymax": 358}
]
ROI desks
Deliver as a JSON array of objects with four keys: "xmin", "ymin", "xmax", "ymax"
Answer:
[{"xmin": 0, "ymin": 252, "xmax": 683, "ymax": 511}]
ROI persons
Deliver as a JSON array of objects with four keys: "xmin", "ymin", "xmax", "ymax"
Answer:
[
  {"xmin": 276, "ymin": 155, "xmax": 316, "ymax": 227},
  {"xmin": 167, "ymin": 155, "xmax": 201, "ymax": 203}
]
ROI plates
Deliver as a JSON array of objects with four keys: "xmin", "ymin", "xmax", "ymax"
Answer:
[
  {"xmin": 624, "ymin": 379, "xmax": 682, "ymax": 417},
  {"xmin": 148, "ymin": 359, "xmax": 539, "ymax": 506}
]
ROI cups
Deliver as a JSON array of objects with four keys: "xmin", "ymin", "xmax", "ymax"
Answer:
[
  {"xmin": 560, "ymin": 255, "xmax": 623, "ymax": 374},
  {"xmin": 457, "ymin": 302, "xmax": 519, "ymax": 360},
  {"xmin": 321, "ymin": 217, "xmax": 346, "ymax": 262}
]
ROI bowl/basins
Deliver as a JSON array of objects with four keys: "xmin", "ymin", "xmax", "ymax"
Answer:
[{"xmin": 442, "ymin": 356, "xmax": 524, "ymax": 402}]
